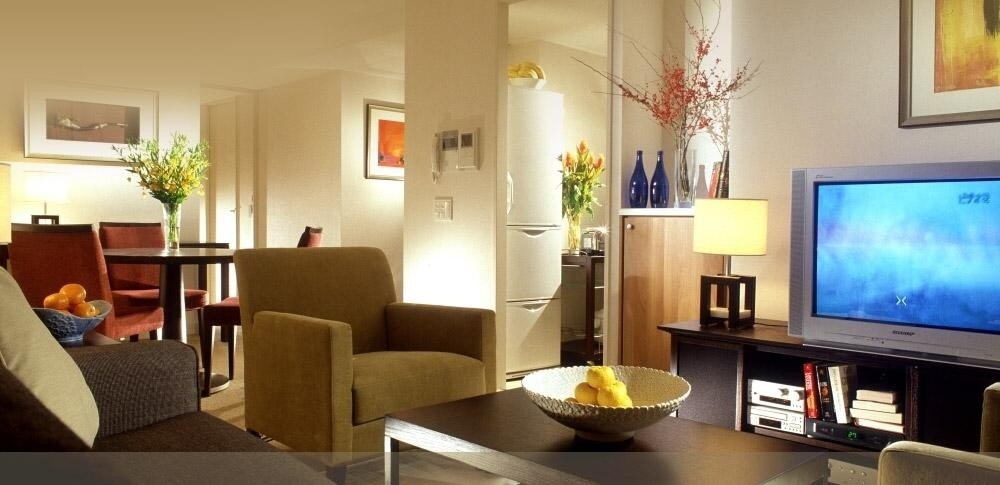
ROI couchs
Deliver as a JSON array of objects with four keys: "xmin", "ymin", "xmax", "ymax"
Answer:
[
  {"xmin": 0, "ymin": 269, "xmax": 336, "ymax": 484},
  {"xmin": 235, "ymin": 248, "xmax": 497, "ymax": 484}
]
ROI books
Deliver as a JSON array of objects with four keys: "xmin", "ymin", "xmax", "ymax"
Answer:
[
  {"xmin": 854, "ymin": 388, "xmax": 894, "ymax": 404},
  {"xmin": 829, "ymin": 364, "xmax": 855, "ymax": 426},
  {"xmin": 853, "ymin": 419, "xmax": 906, "ymax": 434},
  {"xmin": 708, "ymin": 150, "xmax": 730, "ymax": 198},
  {"xmin": 802, "ymin": 361, "xmax": 820, "ymax": 419},
  {"xmin": 850, "ymin": 399, "xmax": 899, "ymax": 413},
  {"xmin": 815, "ymin": 363, "xmax": 836, "ymax": 422},
  {"xmin": 848, "ymin": 408, "xmax": 903, "ymax": 424}
]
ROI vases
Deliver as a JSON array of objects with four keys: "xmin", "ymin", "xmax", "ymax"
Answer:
[
  {"xmin": 165, "ymin": 204, "xmax": 181, "ymax": 254},
  {"xmin": 673, "ymin": 148, "xmax": 696, "ymax": 211},
  {"xmin": 567, "ymin": 209, "xmax": 581, "ymax": 258}
]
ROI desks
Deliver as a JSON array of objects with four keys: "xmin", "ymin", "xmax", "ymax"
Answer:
[{"xmin": 103, "ymin": 247, "xmax": 234, "ymax": 397}]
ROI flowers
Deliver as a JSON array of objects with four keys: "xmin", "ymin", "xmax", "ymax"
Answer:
[
  {"xmin": 110, "ymin": 130, "xmax": 210, "ymax": 243},
  {"xmin": 563, "ymin": 139, "xmax": 607, "ymax": 249},
  {"xmin": 569, "ymin": 1, "xmax": 769, "ymax": 198}
]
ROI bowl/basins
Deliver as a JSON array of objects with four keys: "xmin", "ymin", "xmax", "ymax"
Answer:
[{"xmin": 522, "ymin": 365, "xmax": 691, "ymax": 441}]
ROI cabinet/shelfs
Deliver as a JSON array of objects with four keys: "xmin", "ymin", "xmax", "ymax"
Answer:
[
  {"xmin": 562, "ymin": 255, "xmax": 604, "ymax": 362},
  {"xmin": 659, "ymin": 317, "xmax": 1000, "ymax": 452},
  {"xmin": 617, "ymin": 207, "xmax": 723, "ymax": 371}
]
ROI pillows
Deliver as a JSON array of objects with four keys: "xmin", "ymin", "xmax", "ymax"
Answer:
[{"xmin": 0, "ymin": 267, "xmax": 102, "ymax": 448}]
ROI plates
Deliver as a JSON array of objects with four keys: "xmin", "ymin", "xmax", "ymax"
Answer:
[{"xmin": 31, "ymin": 300, "xmax": 112, "ymax": 342}]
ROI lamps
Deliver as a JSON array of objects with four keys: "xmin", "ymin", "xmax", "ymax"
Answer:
[
  {"xmin": 21, "ymin": 170, "xmax": 59, "ymax": 224},
  {"xmin": 695, "ymin": 200, "xmax": 769, "ymax": 318},
  {"xmin": 0, "ymin": 163, "xmax": 13, "ymax": 267}
]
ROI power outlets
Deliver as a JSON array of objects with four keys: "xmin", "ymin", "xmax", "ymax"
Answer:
[{"xmin": 434, "ymin": 195, "xmax": 454, "ymax": 219}]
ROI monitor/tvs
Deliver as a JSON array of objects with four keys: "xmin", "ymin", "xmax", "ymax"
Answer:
[{"xmin": 787, "ymin": 161, "xmax": 1000, "ymax": 367}]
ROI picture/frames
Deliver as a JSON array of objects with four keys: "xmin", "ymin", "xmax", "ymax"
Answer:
[
  {"xmin": 898, "ymin": 1, "xmax": 1000, "ymax": 130},
  {"xmin": 365, "ymin": 103, "xmax": 406, "ymax": 180},
  {"xmin": 23, "ymin": 77, "xmax": 160, "ymax": 165}
]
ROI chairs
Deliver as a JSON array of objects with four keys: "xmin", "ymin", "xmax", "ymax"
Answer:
[
  {"xmin": 99, "ymin": 221, "xmax": 206, "ymax": 368},
  {"xmin": 11, "ymin": 223, "xmax": 165, "ymax": 342},
  {"xmin": 201, "ymin": 227, "xmax": 323, "ymax": 397}
]
